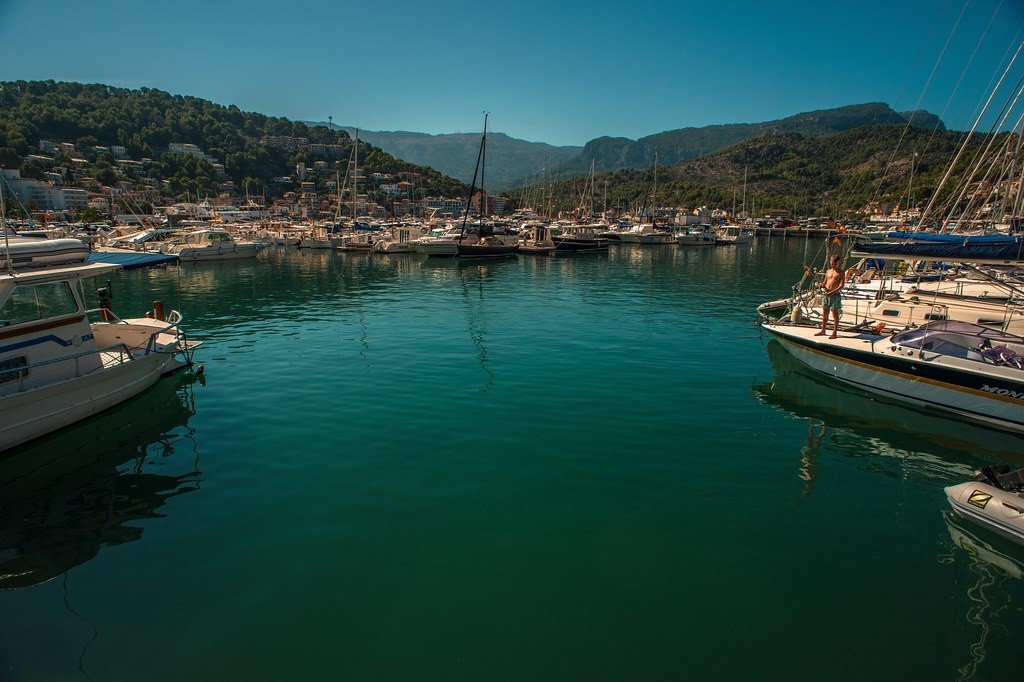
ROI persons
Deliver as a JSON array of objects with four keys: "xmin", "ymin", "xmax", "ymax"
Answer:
[{"xmin": 815, "ymin": 255, "xmax": 845, "ymax": 339}]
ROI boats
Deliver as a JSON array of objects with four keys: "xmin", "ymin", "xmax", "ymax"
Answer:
[
  {"xmin": 0, "ymin": 237, "xmax": 204, "ymax": 453},
  {"xmin": 944, "ymin": 461, "xmax": 1024, "ymax": 547},
  {"xmin": 0, "ymin": 41, "xmax": 1024, "ymax": 440}
]
